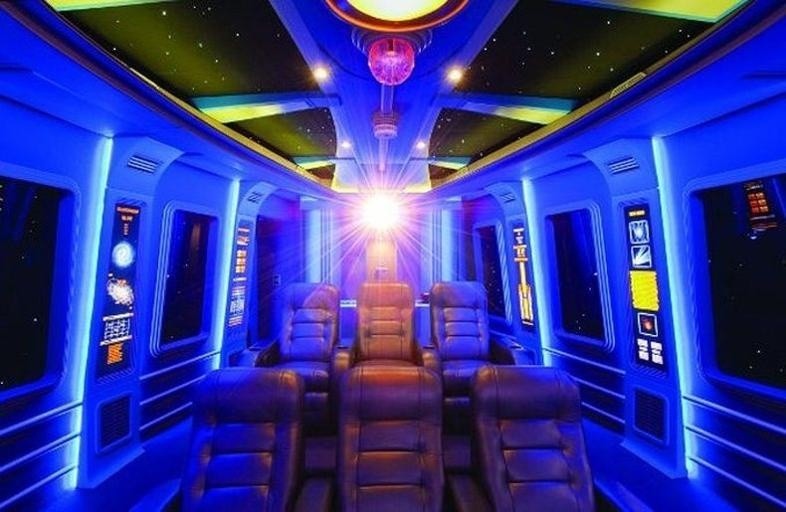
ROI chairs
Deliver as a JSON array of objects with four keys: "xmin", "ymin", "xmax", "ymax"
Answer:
[{"xmin": 130, "ymin": 281, "xmax": 652, "ymax": 512}]
